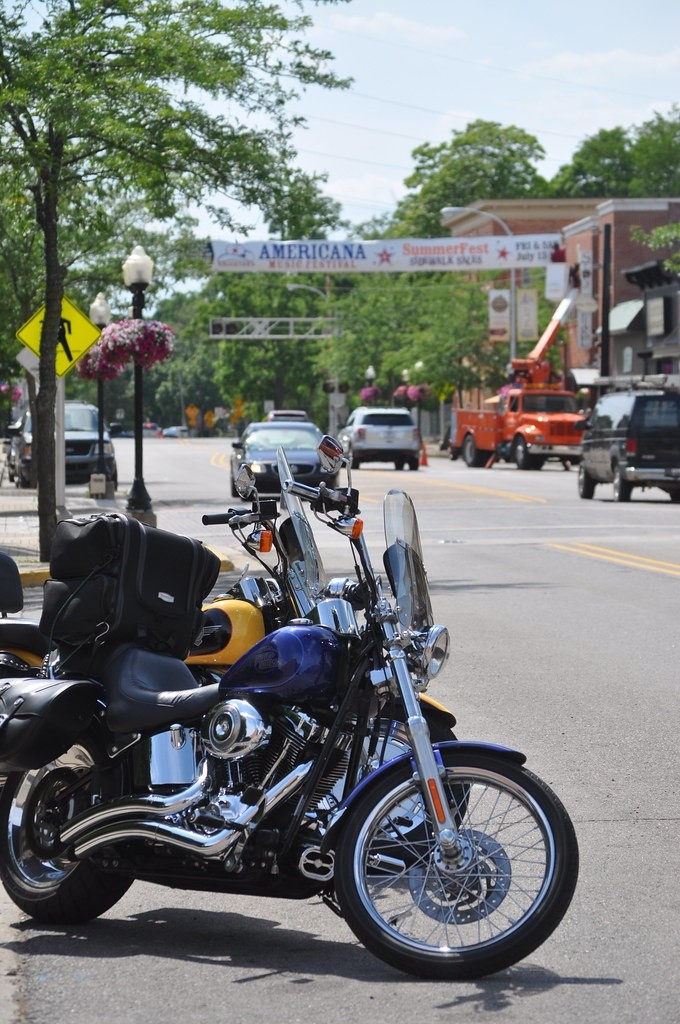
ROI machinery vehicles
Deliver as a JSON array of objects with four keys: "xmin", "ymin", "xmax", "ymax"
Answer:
[{"xmin": 438, "ymin": 261, "xmax": 587, "ymax": 471}]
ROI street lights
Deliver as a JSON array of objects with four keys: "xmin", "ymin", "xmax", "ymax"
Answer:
[
  {"xmin": 121, "ymin": 244, "xmax": 158, "ymax": 529},
  {"xmin": 438, "ymin": 205, "xmax": 517, "ymax": 377},
  {"xmin": 89, "ymin": 289, "xmax": 116, "ymax": 500}
]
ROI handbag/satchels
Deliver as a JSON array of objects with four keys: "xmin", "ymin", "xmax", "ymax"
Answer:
[{"xmin": 39, "ymin": 512, "xmax": 222, "ymax": 660}]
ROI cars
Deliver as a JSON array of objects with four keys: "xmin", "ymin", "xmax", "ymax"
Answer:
[
  {"xmin": 109, "ymin": 421, "xmax": 189, "ymax": 438},
  {"xmin": 3, "ymin": 399, "xmax": 118, "ymax": 489},
  {"xmin": 228, "ymin": 419, "xmax": 341, "ymax": 499}
]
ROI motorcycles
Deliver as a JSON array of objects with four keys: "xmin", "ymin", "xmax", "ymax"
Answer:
[
  {"xmin": 0, "ymin": 432, "xmax": 580, "ymax": 983},
  {"xmin": 0, "ymin": 444, "xmax": 330, "ymax": 680}
]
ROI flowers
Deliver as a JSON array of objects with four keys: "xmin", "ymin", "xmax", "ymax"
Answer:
[
  {"xmin": 73, "ymin": 318, "xmax": 177, "ymax": 378},
  {"xmin": 361, "ymin": 384, "xmax": 431, "ymax": 403},
  {"xmin": 1, "ymin": 385, "xmax": 21, "ymax": 402}
]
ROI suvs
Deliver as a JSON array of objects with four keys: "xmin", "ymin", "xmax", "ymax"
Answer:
[
  {"xmin": 335, "ymin": 406, "xmax": 422, "ymax": 471},
  {"xmin": 574, "ymin": 381, "xmax": 680, "ymax": 503}
]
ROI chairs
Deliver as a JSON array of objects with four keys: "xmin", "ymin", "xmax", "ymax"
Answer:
[{"xmin": 546, "ymin": 400, "xmax": 565, "ymax": 414}]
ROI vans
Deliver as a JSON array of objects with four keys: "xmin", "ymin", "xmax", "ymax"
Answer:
[{"xmin": 261, "ymin": 409, "xmax": 311, "ymax": 421}]
